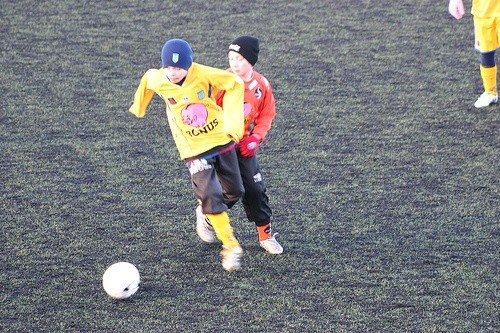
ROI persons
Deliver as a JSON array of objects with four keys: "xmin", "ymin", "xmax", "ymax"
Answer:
[
  {"xmin": 196, "ymin": 35, "xmax": 283, "ymax": 254},
  {"xmin": 129, "ymin": 39, "xmax": 244, "ymax": 272},
  {"xmin": 449, "ymin": 0, "xmax": 500, "ymax": 109}
]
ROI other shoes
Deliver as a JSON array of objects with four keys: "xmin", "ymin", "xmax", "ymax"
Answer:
[
  {"xmin": 259, "ymin": 232, "xmax": 284, "ymax": 254},
  {"xmin": 474, "ymin": 92, "xmax": 498, "ymax": 108},
  {"xmin": 220, "ymin": 246, "xmax": 243, "ymax": 272},
  {"xmin": 195, "ymin": 205, "xmax": 217, "ymax": 243}
]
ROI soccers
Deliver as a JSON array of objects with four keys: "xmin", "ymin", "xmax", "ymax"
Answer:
[{"xmin": 102, "ymin": 262, "xmax": 140, "ymax": 300}]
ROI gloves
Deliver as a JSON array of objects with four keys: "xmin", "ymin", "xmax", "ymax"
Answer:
[{"xmin": 239, "ymin": 135, "xmax": 261, "ymax": 158}]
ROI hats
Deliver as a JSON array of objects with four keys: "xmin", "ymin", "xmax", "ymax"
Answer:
[
  {"xmin": 227, "ymin": 35, "xmax": 260, "ymax": 65},
  {"xmin": 161, "ymin": 39, "xmax": 193, "ymax": 70}
]
